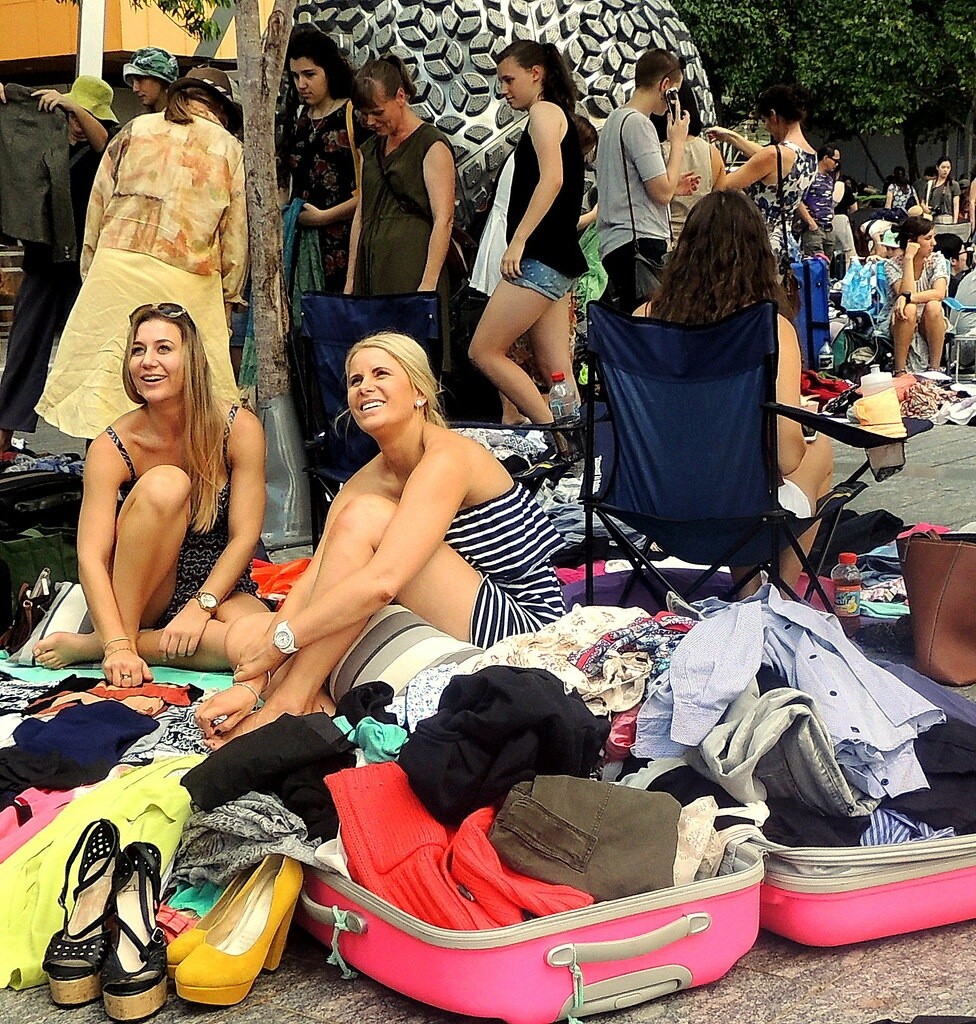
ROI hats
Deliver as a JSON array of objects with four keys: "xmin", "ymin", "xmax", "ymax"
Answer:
[
  {"xmin": 123, "ymin": 47, "xmax": 179, "ymax": 86},
  {"xmin": 61, "ymin": 75, "xmax": 120, "ymax": 127},
  {"xmin": 877, "ymin": 228, "xmax": 900, "ymax": 248},
  {"xmin": 167, "ymin": 68, "xmax": 243, "ymax": 135}
]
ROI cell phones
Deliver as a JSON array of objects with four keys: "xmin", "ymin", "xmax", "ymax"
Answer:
[{"xmin": 666, "ymin": 86, "xmax": 684, "ymax": 123}]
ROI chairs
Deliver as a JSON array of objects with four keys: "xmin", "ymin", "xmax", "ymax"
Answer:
[
  {"xmin": 877, "ymin": 261, "xmax": 976, "ymax": 387},
  {"xmin": 287, "ymin": 290, "xmax": 583, "ymax": 556},
  {"xmin": 846, "ymin": 257, "xmax": 893, "ymax": 351},
  {"xmin": 582, "ymin": 300, "xmax": 932, "ymax": 613}
]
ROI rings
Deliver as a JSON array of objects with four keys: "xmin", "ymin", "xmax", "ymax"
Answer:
[{"xmin": 123, "ymin": 674, "xmax": 131, "ymax": 679}]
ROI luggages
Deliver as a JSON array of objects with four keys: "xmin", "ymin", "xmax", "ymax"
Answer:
[{"xmin": 297, "ymin": 838, "xmax": 976, "ymax": 1024}]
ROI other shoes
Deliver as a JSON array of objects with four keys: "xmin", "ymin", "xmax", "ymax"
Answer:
[
  {"xmin": 154, "ymin": 889, "xmax": 200, "ymax": 944},
  {"xmin": 924, "ymin": 366, "xmax": 941, "ymax": 373},
  {"xmin": 892, "ymin": 369, "xmax": 907, "ymax": 378}
]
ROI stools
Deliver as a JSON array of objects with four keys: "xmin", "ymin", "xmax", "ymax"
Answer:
[{"xmin": 945, "ymin": 335, "xmax": 976, "ymax": 384}]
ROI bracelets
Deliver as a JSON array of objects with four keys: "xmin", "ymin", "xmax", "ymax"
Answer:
[
  {"xmin": 103, "ymin": 647, "xmax": 131, "ymax": 662},
  {"xmin": 104, "ymin": 637, "xmax": 130, "ymax": 650},
  {"xmin": 233, "ymin": 682, "xmax": 259, "ymax": 706},
  {"xmin": 262, "ymin": 670, "xmax": 270, "ymax": 694}
]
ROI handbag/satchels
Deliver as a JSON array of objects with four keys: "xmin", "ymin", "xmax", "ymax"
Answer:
[
  {"xmin": 923, "ymin": 180, "xmax": 933, "ymax": 221},
  {"xmin": 445, "ymin": 237, "xmax": 467, "ymax": 283},
  {"xmin": 634, "ymin": 250, "xmax": 673, "ymax": 300},
  {"xmin": 906, "ymin": 185, "xmax": 917, "ymax": 211},
  {"xmin": 778, "ymin": 269, "xmax": 801, "ymax": 316},
  {"xmin": 895, "ymin": 529, "xmax": 976, "ymax": 687}
]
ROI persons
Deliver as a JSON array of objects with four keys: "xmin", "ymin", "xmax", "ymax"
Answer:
[
  {"xmin": 30, "ymin": 300, "xmax": 271, "ymax": 673},
  {"xmin": 193, "ymin": 332, "xmax": 566, "ymax": 752},
  {"xmin": 1, "ymin": 32, "xmax": 976, "ymax": 602}
]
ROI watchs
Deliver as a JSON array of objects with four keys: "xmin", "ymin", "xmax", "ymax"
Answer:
[
  {"xmin": 273, "ymin": 621, "xmax": 299, "ymax": 655},
  {"xmin": 192, "ymin": 592, "xmax": 219, "ymax": 615}
]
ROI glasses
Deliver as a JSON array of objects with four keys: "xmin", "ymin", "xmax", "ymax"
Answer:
[
  {"xmin": 957, "ymin": 246, "xmax": 974, "ymax": 256},
  {"xmin": 824, "ymin": 154, "xmax": 842, "ymax": 165},
  {"xmin": 129, "ymin": 301, "xmax": 195, "ymax": 328}
]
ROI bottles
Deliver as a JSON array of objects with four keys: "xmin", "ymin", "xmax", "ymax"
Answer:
[
  {"xmin": 549, "ymin": 371, "xmax": 584, "ymax": 461},
  {"xmin": 818, "ymin": 336, "xmax": 836, "ymax": 377},
  {"xmin": 830, "ymin": 552, "xmax": 861, "ymax": 638},
  {"xmin": 861, "ymin": 363, "xmax": 906, "ymax": 469}
]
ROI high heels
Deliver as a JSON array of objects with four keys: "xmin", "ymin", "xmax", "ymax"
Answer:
[
  {"xmin": 42, "ymin": 819, "xmax": 120, "ymax": 1010},
  {"xmin": 175, "ymin": 851, "xmax": 304, "ymax": 1006},
  {"xmin": 100, "ymin": 842, "xmax": 168, "ymax": 1024},
  {"xmin": 166, "ymin": 861, "xmax": 263, "ymax": 979}
]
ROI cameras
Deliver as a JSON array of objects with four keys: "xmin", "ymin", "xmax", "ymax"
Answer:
[{"xmin": 931, "ymin": 206, "xmax": 941, "ymax": 213}]
